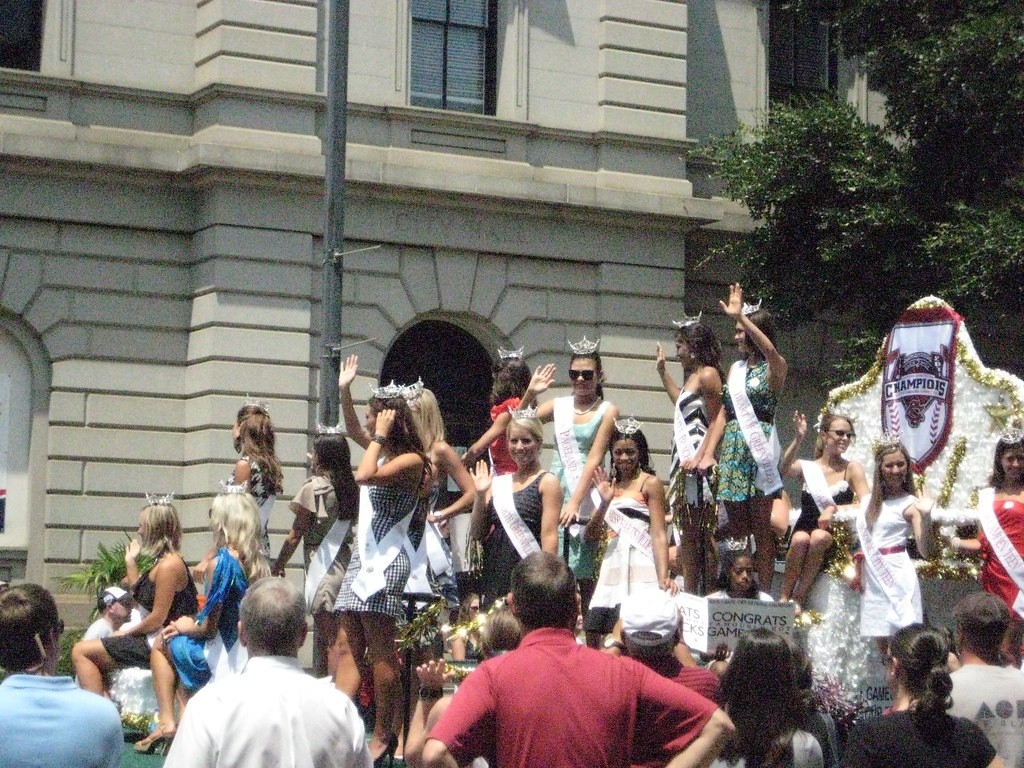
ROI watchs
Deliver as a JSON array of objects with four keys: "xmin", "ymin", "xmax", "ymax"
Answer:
[{"xmin": 419, "ymin": 687, "xmax": 443, "ymax": 701}]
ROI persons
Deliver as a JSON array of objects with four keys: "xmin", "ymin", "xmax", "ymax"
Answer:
[
  {"xmin": 0, "ymin": 281, "xmax": 787, "ymax": 768},
  {"xmin": 420, "ymin": 549, "xmax": 734, "ymax": 768},
  {"xmin": 856, "ymin": 442, "xmax": 937, "ymax": 701},
  {"xmin": 780, "ymin": 410, "xmax": 870, "ymax": 612},
  {"xmin": 715, "ymin": 627, "xmax": 823, "ymax": 768},
  {"xmin": 835, "ymin": 622, "xmax": 1004, "ymax": 768},
  {"xmin": 937, "ymin": 427, "xmax": 1024, "ymax": 667},
  {"xmin": 193, "ymin": 405, "xmax": 280, "ymax": 585},
  {"xmin": 946, "ymin": 594, "xmax": 1024, "ymax": 768},
  {"xmin": 468, "ymin": 416, "xmax": 564, "ymax": 608}
]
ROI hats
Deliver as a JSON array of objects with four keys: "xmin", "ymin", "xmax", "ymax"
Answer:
[
  {"xmin": 953, "ymin": 591, "xmax": 1010, "ymax": 639},
  {"xmin": 618, "ymin": 589, "xmax": 678, "ymax": 646},
  {"xmin": 96, "ymin": 585, "xmax": 132, "ymax": 612}
]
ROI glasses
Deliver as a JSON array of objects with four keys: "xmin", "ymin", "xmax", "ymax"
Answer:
[
  {"xmin": 881, "ymin": 654, "xmax": 893, "ymax": 667},
  {"xmin": 825, "ymin": 429, "xmax": 853, "ymax": 439},
  {"xmin": 569, "ymin": 369, "xmax": 596, "ymax": 381},
  {"xmin": 44, "ymin": 619, "xmax": 64, "ymax": 636},
  {"xmin": 209, "ymin": 509, "xmax": 214, "ymax": 518}
]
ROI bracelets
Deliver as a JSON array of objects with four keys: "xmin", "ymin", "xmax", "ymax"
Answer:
[
  {"xmin": 374, "ymin": 433, "xmax": 387, "ymax": 446},
  {"xmin": 952, "ymin": 537, "xmax": 960, "ymax": 548}
]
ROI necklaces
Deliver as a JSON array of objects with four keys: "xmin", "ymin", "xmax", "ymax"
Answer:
[{"xmin": 574, "ymin": 398, "xmax": 600, "ymax": 415}]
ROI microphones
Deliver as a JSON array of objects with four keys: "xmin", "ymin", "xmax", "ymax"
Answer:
[{"xmin": 400, "ymin": 592, "xmax": 440, "ymax": 603}]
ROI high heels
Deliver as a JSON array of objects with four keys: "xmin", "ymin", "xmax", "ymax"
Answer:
[
  {"xmin": 134, "ymin": 720, "xmax": 176, "ymax": 755},
  {"xmin": 370, "ymin": 731, "xmax": 399, "ymax": 768}
]
